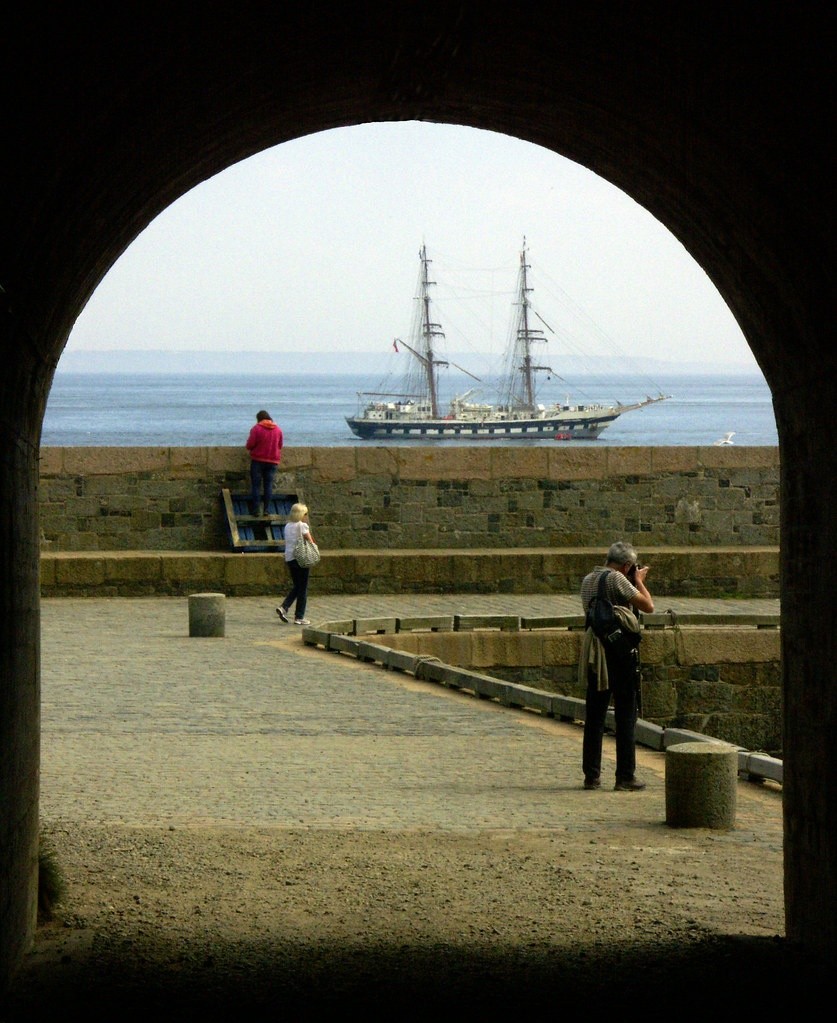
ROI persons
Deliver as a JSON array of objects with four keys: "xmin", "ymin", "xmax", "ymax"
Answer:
[
  {"xmin": 276, "ymin": 503, "xmax": 320, "ymax": 625},
  {"xmin": 245, "ymin": 410, "xmax": 283, "ymax": 517},
  {"xmin": 576, "ymin": 541, "xmax": 654, "ymax": 792}
]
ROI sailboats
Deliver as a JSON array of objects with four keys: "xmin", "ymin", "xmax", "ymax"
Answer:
[{"xmin": 343, "ymin": 234, "xmax": 673, "ymax": 441}]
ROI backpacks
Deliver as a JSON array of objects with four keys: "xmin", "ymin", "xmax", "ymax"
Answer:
[{"xmin": 589, "ymin": 571, "xmax": 641, "ymax": 654}]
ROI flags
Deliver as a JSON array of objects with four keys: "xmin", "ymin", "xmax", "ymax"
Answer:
[{"xmin": 393, "ymin": 341, "xmax": 399, "ymax": 352}]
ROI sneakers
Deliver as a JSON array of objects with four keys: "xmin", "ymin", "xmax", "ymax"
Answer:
[
  {"xmin": 276, "ymin": 606, "xmax": 289, "ymax": 623},
  {"xmin": 294, "ymin": 618, "xmax": 309, "ymax": 625}
]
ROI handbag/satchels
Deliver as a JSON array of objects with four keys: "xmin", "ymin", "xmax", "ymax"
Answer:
[{"xmin": 295, "ymin": 523, "xmax": 320, "ymax": 570}]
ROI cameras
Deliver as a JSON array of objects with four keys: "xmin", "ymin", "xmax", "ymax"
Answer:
[{"xmin": 628, "ymin": 563, "xmax": 643, "ymax": 576}]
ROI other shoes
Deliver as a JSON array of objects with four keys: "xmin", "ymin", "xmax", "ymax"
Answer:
[
  {"xmin": 582, "ymin": 774, "xmax": 600, "ymax": 789},
  {"xmin": 252, "ymin": 511, "xmax": 269, "ymax": 517},
  {"xmin": 614, "ymin": 777, "xmax": 650, "ymax": 791}
]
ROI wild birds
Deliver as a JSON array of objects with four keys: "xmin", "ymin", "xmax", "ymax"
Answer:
[{"xmin": 714, "ymin": 432, "xmax": 736, "ymax": 447}]
